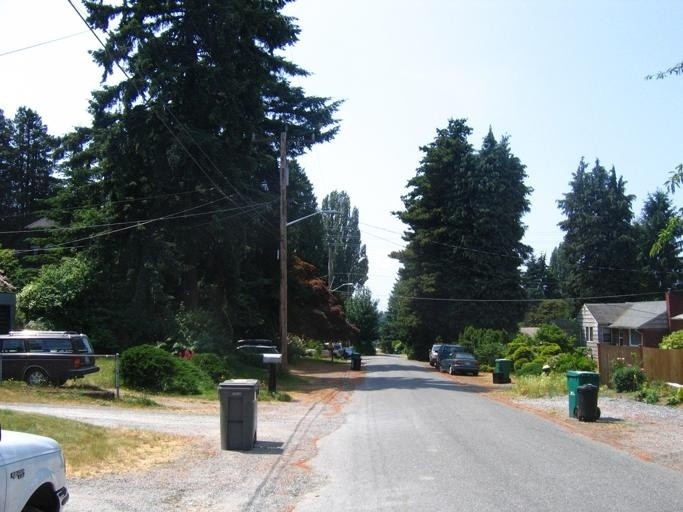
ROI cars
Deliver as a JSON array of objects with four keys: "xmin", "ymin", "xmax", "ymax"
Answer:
[
  {"xmin": 428, "ymin": 342, "xmax": 479, "ymax": 377},
  {"xmin": 222, "ymin": 338, "xmax": 282, "ymax": 377},
  {"xmin": 306, "ymin": 342, "xmax": 352, "ymax": 357},
  {"xmin": 0, "ymin": 430, "xmax": 69, "ymax": 512}
]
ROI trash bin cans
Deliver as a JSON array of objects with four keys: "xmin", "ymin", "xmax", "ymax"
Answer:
[
  {"xmin": 495, "ymin": 358, "xmax": 513, "ymax": 383},
  {"xmin": 566, "ymin": 370, "xmax": 600, "ymax": 422},
  {"xmin": 217, "ymin": 379, "xmax": 259, "ymax": 450},
  {"xmin": 351, "ymin": 352, "xmax": 362, "ymax": 370}
]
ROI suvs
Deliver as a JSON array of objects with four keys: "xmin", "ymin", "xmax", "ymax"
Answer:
[{"xmin": 0, "ymin": 331, "xmax": 99, "ymax": 387}]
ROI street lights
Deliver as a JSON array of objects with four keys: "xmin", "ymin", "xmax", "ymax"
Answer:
[
  {"xmin": 279, "ymin": 210, "xmax": 343, "ymax": 373},
  {"xmin": 328, "ymin": 282, "xmax": 354, "ymax": 292}
]
